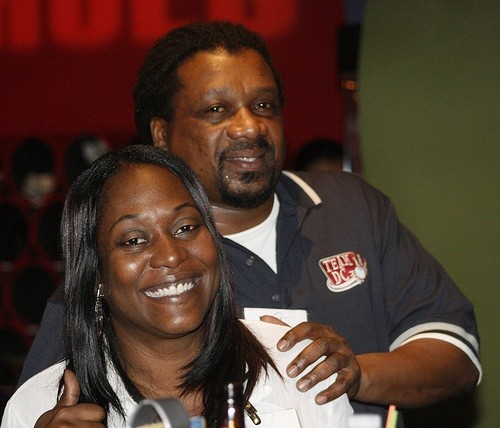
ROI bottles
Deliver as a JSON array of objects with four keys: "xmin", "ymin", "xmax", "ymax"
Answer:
[{"xmin": 211, "ymin": 381, "xmax": 246, "ymax": 428}]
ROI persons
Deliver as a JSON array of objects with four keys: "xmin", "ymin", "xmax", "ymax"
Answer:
[
  {"xmin": 17, "ymin": 20, "xmax": 481, "ymax": 427},
  {"xmin": 2, "ymin": 145, "xmax": 356, "ymax": 428},
  {"xmin": 290, "ymin": 139, "xmax": 354, "ymax": 174}
]
